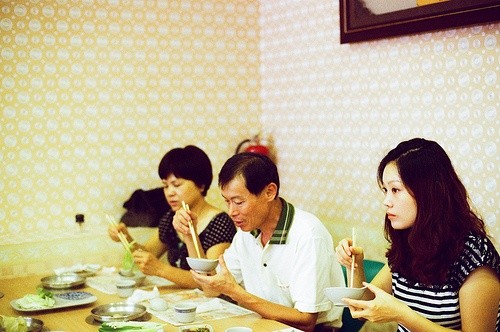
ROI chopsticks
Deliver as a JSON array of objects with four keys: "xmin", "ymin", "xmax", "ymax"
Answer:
[
  {"xmin": 182, "ymin": 201, "xmax": 203, "ymax": 260},
  {"xmin": 347, "ymin": 227, "xmax": 356, "ymax": 288},
  {"xmin": 105, "ymin": 214, "xmax": 135, "ymax": 258}
]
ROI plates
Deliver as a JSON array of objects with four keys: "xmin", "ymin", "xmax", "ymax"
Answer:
[
  {"xmin": 10, "ymin": 290, "xmax": 97, "ymax": 312},
  {"xmin": 90, "ymin": 301, "xmax": 146, "ymax": 324},
  {"xmin": 22, "ymin": 318, "xmax": 44, "ymax": 332},
  {"xmin": 40, "ymin": 274, "xmax": 87, "ymax": 289}
]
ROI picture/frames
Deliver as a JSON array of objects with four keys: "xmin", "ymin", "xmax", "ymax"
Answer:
[{"xmin": 339, "ymin": 0, "xmax": 500, "ymax": 45}]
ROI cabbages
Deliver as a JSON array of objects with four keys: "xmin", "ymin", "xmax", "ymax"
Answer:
[
  {"xmin": 0, "ymin": 315, "xmax": 29, "ymax": 332},
  {"xmin": 15, "ymin": 293, "xmax": 56, "ymax": 308},
  {"xmin": 122, "ymin": 240, "xmax": 138, "ymax": 276}
]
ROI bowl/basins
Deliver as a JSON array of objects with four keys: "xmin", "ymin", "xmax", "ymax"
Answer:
[
  {"xmin": 322, "ymin": 286, "xmax": 367, "ymax": 306},
  {"xmin": 178, "ymin": 324, "xmax": 213, "ymax": 332},
  {"xmin": 185, "ymin": 257, "xmax": 218, "ymax": 274},
  {"xmin": 117, "ymin": 274, "xmax": 147, "ymax": 286},
  {"xmin": 226, "ymin": 327, "xmax": 253, "ymax": 332}
]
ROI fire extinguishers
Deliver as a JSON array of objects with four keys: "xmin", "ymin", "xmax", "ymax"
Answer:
[{"xmin": 235, "ymin": 128, "xmax": 270, "ymax": 157}]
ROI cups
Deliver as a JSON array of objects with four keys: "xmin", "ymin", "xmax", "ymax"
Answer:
[
  {"xmin": 173, "ymin": 302, "xmax": 196, "ymax": 323},
  {"xmin": 113, "ymin": 278, "xmax": 137, "ymax": 297}
]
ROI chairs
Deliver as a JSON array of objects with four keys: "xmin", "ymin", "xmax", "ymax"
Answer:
[{"xmin": 339, "ymin": 260, "xmax": 385, "ymax": 332}]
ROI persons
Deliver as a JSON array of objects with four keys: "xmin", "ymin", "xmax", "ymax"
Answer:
[
  {"xmin": 108, "ymin": 145, "xmax": 237, "ymax": 304},
  {"xmin": 173, "ymin": 151, "xmax": 347, "ymax": 332},
  {"xmin": 335, "ymin": 137, "xmax": 500, "ymax": 332}
]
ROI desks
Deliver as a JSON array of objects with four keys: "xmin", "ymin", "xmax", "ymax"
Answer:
[{"xmin": 0, "ymin": 268, "xmax": 305, "ymax": 331}]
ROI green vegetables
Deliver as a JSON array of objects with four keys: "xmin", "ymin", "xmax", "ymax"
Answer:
[
  {"xmin": 98, "ymin": 323, "xmax": 169, "ymax": 332},
  {"xmin": 36, "ymin": 288, "xmax": 54, "ymax": 299}
]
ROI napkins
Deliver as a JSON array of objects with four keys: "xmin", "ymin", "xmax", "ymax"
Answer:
[
  {"xmin": 188, "ymin": 298, "xmax": 222, "ymax": 313},
  {"xmin": 125, "ymin": 285, "xmax": 161, "ymax": 303}
]
